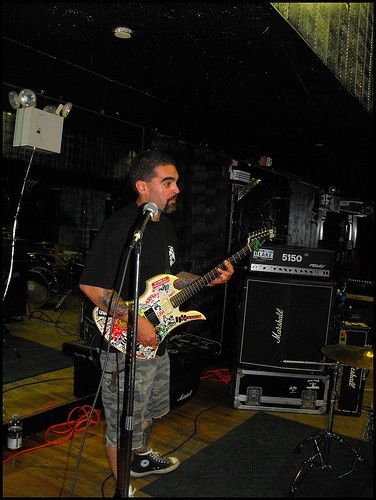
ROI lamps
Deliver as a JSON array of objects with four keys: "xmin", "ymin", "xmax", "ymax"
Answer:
[
  {"xmin": 329, "ymin": 186, "xmax": 336, "ymax": 194},
  {"xmin": 8, "ymin": 89, "xmax": 36, "ymax": 109},
  {"xmin": 43, "ymin": 102, "xmax": 73, "ymax": 118},
  {"xmin": 112, "ymin": 27, "xmax": 134, "ymax": 39}
]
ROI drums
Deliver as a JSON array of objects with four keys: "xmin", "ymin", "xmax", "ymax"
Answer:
[
  {"xmin": 26, "ymin": 267, "xmax": 57, "ymax": 308},
  {"xmin": 25, "ymin": 252, "xmax": 51, "ymax": 267}
]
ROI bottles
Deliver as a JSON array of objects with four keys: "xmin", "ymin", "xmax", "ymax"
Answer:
[{"xmin": 6, "ymin": 414, "xmax": 22, "ymax": 452}]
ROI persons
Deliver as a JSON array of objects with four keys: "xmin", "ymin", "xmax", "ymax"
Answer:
[{"xmin": 79, "ymin": 151, "xmax": 234, "ymax": 497}]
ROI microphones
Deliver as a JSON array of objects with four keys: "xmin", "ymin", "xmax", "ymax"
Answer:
[{"xmin": 129, "ymin": 201, "xmax": 158, "ymax": 249}]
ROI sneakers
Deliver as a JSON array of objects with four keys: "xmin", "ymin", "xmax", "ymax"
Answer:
[{"xmin": 130, "ymin": 447, "xmax": 181, "ymax": 477}]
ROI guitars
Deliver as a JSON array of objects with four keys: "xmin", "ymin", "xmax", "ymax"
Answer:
[{"xmin": 92, "ymin": 228, "xmax": 274, "ymax": 359}]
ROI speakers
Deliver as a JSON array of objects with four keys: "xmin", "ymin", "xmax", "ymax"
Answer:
[
  {"xmin": 0, "ymin": 259, "xmax": 27, "ymax": 315},
  {"xmin": 237, "ymin": 275, "xmax": 337, "ymax": 373},
  {"xmin": 339, "ymin": 278, "xmax": 376, "ymax": 328}
]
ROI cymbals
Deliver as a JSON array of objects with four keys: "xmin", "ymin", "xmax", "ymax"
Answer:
[
  {"xmin": 34, "ymin": 242, "xmax": 56, "ymax": 245},
  {"xmin": 321, "ymin": 344, "xmax": 373, "ymax": 370},
  {"xmin": 47, "ymin": 254, "xmax": 74, "ymax": 258}
]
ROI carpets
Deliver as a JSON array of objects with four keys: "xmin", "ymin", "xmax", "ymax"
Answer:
[
  {"xmin": 136, "ymin": 411, "xmax": 376, "ymax": 500},
  {"xmin": 0, "ymin": 333, "xmax": 74, "ymax": 384}
]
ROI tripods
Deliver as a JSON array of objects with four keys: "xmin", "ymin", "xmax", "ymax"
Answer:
[{"xmin": 288, "ymin": 368, "xmax": 376, "ymax": 494}]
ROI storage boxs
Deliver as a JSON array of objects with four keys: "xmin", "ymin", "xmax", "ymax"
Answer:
[{"xmin": 234, "ymin": 367, "xmax": 329, "ymax": 414}]
